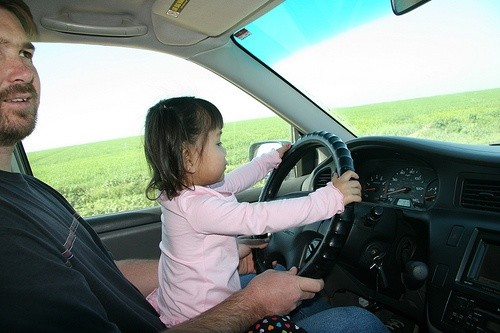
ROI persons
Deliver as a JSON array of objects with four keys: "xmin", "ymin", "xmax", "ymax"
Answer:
[
  {"xmin": 145, "ymin": 97, "xmax": 362, "ymax": 333},
  {"xmin": 0, "ymin": 0, "xmax": 390, "ymax": 333}
]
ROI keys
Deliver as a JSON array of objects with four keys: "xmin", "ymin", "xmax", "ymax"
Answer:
[{"xmin": 368, "ymin": 250, "xmax": 387, "ymax": 273}]
